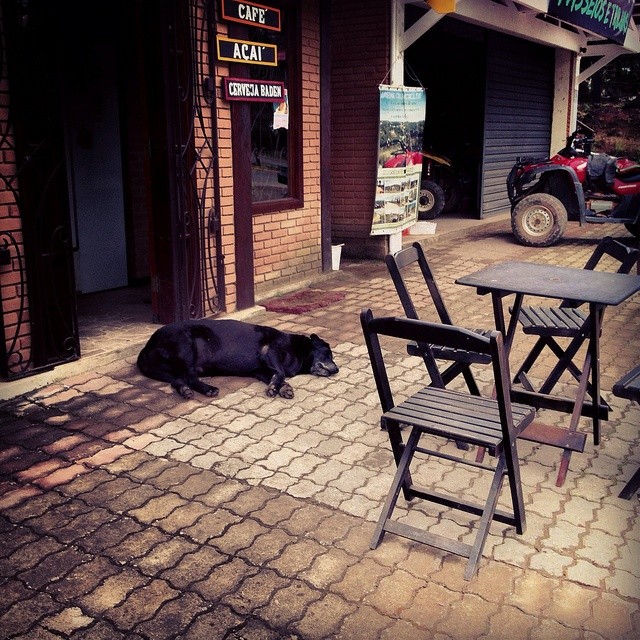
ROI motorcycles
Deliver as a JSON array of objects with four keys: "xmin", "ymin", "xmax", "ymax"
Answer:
[
  {"xmin": 507, "ymin": 129, "xmax": 640, "ymax": 247},
  {"xmin": 417, "ymin": 147, "xmax": 456, "ymax": 219},
  {"xmin": 381, "ymin": 139, "xmax": 423, "ymax": 167}
]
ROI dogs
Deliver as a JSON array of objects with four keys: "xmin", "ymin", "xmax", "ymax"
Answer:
[{"xmin": 136, "ymin": 318, "xmax": 339, "ymax": 399}]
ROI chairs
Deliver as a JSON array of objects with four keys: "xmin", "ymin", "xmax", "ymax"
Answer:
[
  {"xmin": 384, "ymin": 243, "xmax": 505, "ymax": 451},
  {"xmin": 359, "ymin": 309, "xmax": 535, "ymax": 582},
  {"xmin": 510, "ymin": 236, "xmax": 638, "ymax": 444},
  {"xmin": 612, "ymin": 360, "xmax": 640, "ymax": 498}
]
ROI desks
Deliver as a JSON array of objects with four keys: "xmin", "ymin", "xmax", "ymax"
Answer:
[{"xmin": 454, "ymin": 261, "xmax": 639, "ymax": 486}]
